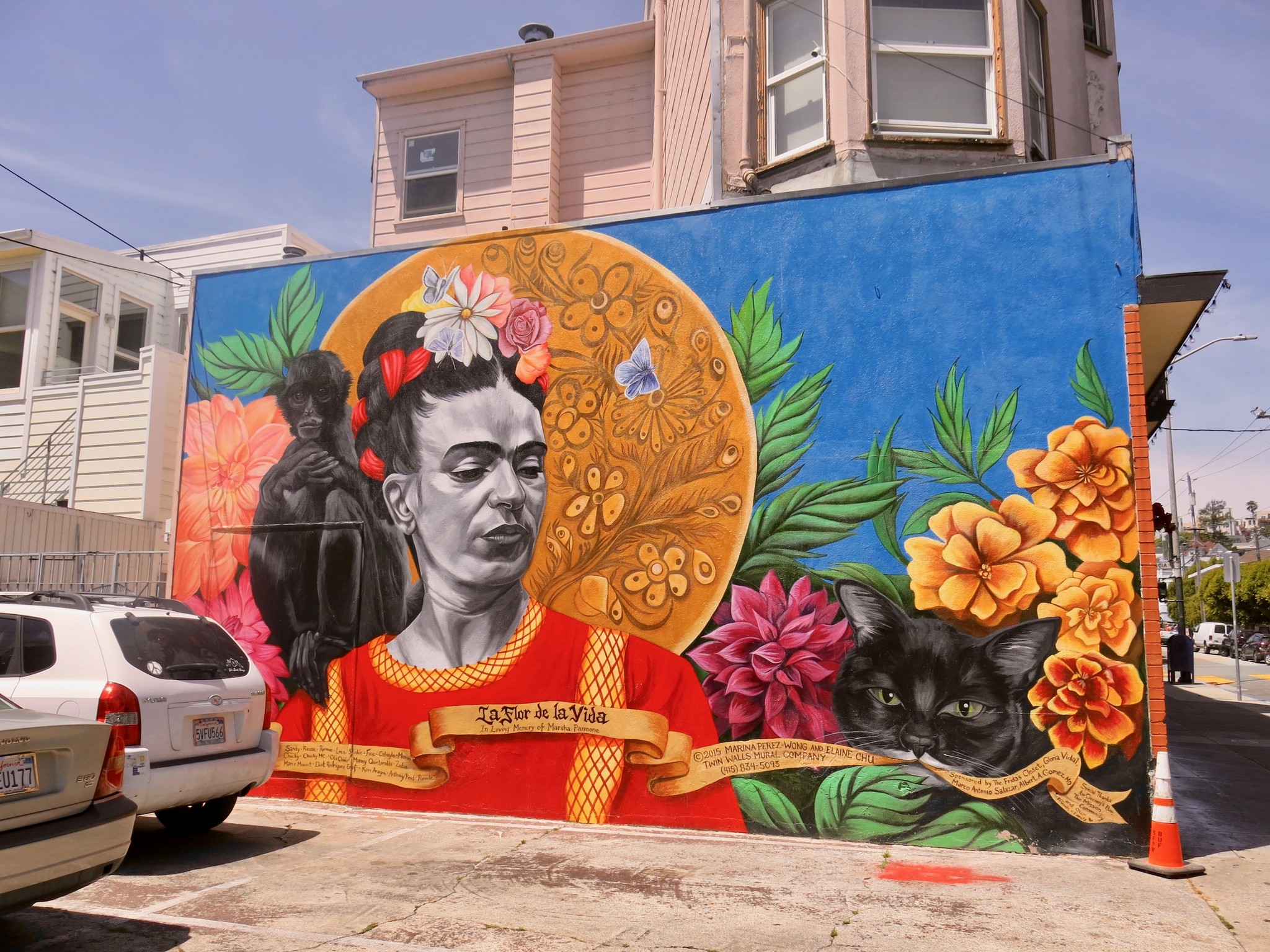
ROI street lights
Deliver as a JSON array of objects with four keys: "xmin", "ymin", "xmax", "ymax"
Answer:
[{"xmin": 1163, "ymin": 333, "xmax": 1260, "ymax": 683}]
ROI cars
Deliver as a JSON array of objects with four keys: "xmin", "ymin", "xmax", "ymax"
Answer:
[
  {"xmin": 0, "ymin": 694, "xmax": 137, "ymax": 915},
  {"xmin": 1264, "ymin": 644, "xmax": 1270, "ymax": 665},
  {"xmin": 1239, "ymin": 633, "xmax": 1270, "ymax": 663}
]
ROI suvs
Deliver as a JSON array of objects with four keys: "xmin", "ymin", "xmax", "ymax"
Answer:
[
  {"xmin": 1, "ymin": 589, "xmax": 281, "ymax": 838},
  {"xmin": 1219, "ymin": 628, "xmax": 1263, "ymax": 658}
]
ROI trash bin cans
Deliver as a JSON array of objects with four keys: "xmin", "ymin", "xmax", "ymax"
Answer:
[{"xmin": 1167, "ymin": 634, "xmax": 1193, "ymax": 673}]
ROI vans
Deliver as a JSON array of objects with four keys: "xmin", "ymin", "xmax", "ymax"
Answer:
[{"xmin": 1192, "ymin": 622, "xmax": 1234, "ymax": 654}]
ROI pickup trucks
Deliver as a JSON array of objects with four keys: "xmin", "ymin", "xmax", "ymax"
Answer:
[{"xmin": 1160, "ymin": 628, "xmax": 1190, "ymax": 645}]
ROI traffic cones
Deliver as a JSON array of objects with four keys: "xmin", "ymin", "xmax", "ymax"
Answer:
[{"xmin": 1125, "ymin": 751, "xmax": 1206, "ymax": 878}]
